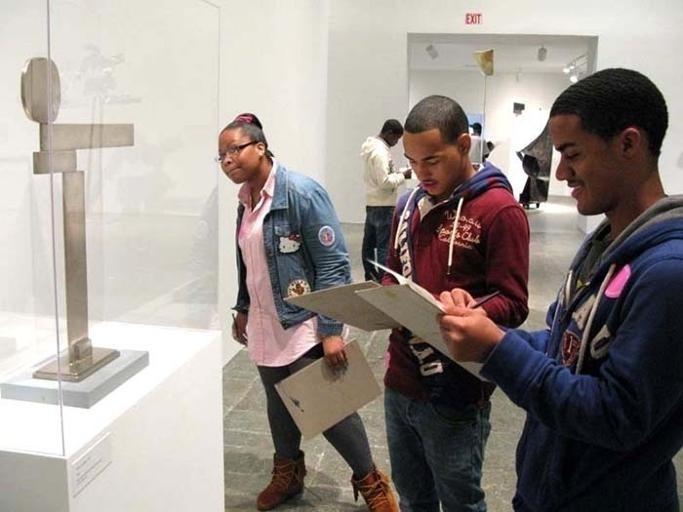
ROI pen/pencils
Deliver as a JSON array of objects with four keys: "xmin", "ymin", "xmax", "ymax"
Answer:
[
  {"xmin": 471, "ymin": 290, "xmax": 501, "ymax": 308},
  {"xmin": 370, "ymin": 272, "xmax": 378, "ymax": 282},
  {"xmin": 232, "ymin": 313, "xmax": 238, "ymax": 337}
]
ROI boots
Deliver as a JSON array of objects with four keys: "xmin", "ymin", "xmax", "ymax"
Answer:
[
  {"xmin": 257, "ymin": 450, "xmax": 322, "ymax": 511},
  {"xmin": 351, "ymin": 462, "xmax": 400, "ymax": 512}
]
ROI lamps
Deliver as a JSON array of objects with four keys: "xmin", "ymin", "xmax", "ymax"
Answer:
[{"xmin": 560, "ymin": 52, "xmax": 590, "ymax": 84}]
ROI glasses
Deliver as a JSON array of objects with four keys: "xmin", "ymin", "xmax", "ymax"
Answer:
[{"xmin": 215, "ymin": 140, "xmax": 261, "ymax": 163}]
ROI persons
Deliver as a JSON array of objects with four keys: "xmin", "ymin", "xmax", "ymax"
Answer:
[
  {"xmin": 360, "ymin": 118, "xmax": 412, "ymax": 285},
  {"xmin": 436, "ymin": 66, "xmax": 682, "ymax": 512},
  {"xmin": 217, "ymin": 113, "xmax": 398, "ymax": 511},
  {"xmin": 468, "ymin": 121, "xmax": 490, "ymax": 166},
  {"xmin": 377, "ymin": 92, "xmax": 533, "ymax": 512}
]
